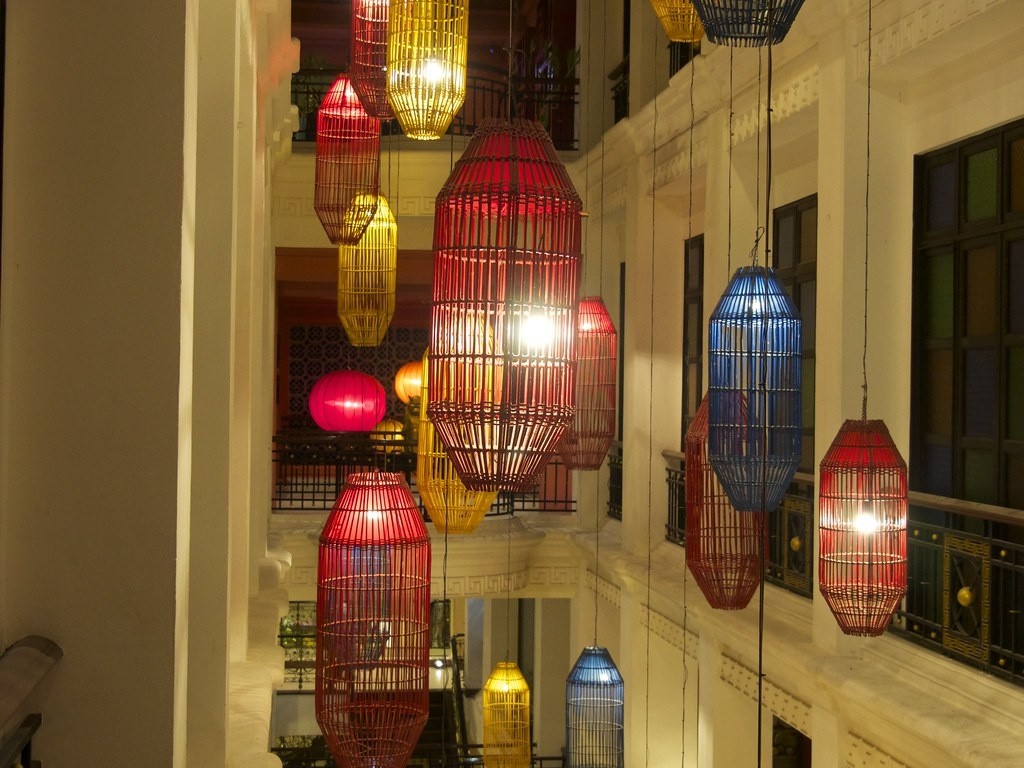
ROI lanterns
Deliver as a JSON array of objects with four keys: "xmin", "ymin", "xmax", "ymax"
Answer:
[
  {"xmin": 394, "ymin": 361, "xmax": 424, "ymax": 404},
  {"xmin": 309, "ymin": 370, "xmax": 386, "ymax": 432},
  {"xmin": 370, "ymin": 420, "xmax": 405, "ymax": 455}
]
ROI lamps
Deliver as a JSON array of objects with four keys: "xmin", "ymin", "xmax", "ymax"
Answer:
[
  {"xmin": 339, "ymin": 191, "xmax": 398, "ymax": 345},
  {"xmin": 395, "ymin": 362, "xmax": 425, "ymax": 407},
  {"xmin": 428, "ymin": 116, "xmax": 583, "ymax": 493},
  {"xmin": 483, "ymin": 661, "xmax": 531, "ymax": 767},
  {"xmin": 707, "ymin": 265, "xmax": 802, "ymax": 512},
  {"xmin": 316, "ymin": 472, "xmax": 432, "ymax": 767},
  {"xmin": 310, "ymin": 366, "xmax": 388, "ymax": 435},
  {"xmin": 555, "ymin": 297, "xmax": 618, "ymax": 469},
  {"xmin": 313, "ymin": 72, "xmax": 380, "ymax": 245},
  {"xmin": 651, "ymin": 0, "xmax": 705, "ymax": 42},
  {"xmin": 388, "ymin": 0, "xmax": 468, "ymax": 143},
  {"xmin": 564, "ymin": 645, "xmax": 624, "ymax": 767},
  {"xmin": 685, "ymin": 389, "xmax": 770, "ymax": 609},
  {"xmin": 416, "ymin": 316, "xmax": 504, "ymax": 532},
  {"xmin": 692, "ymin": 0, "xmax": 805, "ymax": 47},
  {"xmin": 349, "ymin": 1, "xmax": 396, "ymax": 119},
  {"xmin": 818, "ymin": 418, "xmax": 907, "ymax": 637}
]
